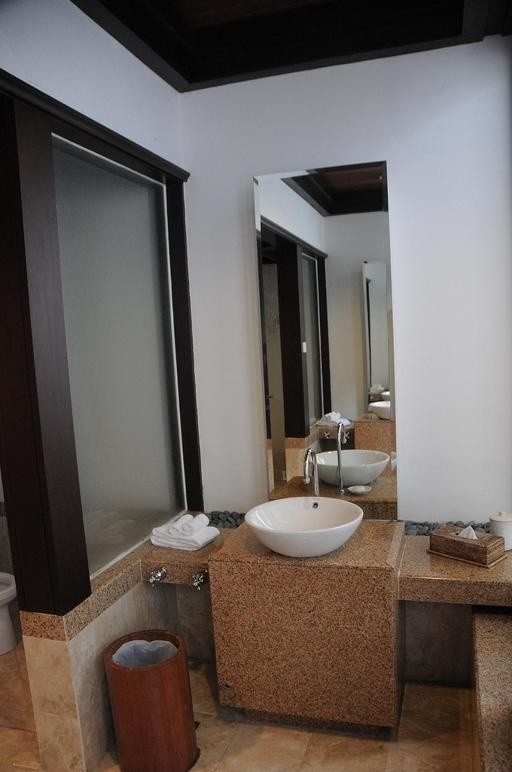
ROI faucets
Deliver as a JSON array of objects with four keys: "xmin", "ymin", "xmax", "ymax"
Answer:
[
  {"xmin": 302, "ymin": 448, "xmax": 321, "ymax": 498},
  {"xmin": 336, "ymin": 422, "xmax": 347, "ymax": 496}
]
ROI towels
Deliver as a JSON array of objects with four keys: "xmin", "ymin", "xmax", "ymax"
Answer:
[
  {"xmin": 316, "ymin": 410, "xmax": 351, "ymax": 427},
  {"xmin": 151, "ymin": 513, "xmax": 220, "ymax": 552},
  {"xmin": 369, "ymin": 383, "xmax": 384, "ymax": 394}
]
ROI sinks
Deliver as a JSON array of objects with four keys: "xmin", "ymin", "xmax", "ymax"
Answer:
[
  {"xmin": 368, "ymin": 400, "xmax": 390, "ymax": 421},
  {"xmin": 245, "ymin": 497, "xmax": 364, "ymax": 561},
  {"xmin": 313, "ymin": 449, "xmax": 391, "ymax": 488}
]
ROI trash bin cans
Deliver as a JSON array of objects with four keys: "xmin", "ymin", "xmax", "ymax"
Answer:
[{"xmin": 103, "ymin": 629, "xmax": 201, "ymax": 770}]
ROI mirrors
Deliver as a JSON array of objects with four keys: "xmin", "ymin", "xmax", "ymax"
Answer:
[
  {"xmin": 252, "ymin": 160, "xmax": 400, "ymax": 521},
  {"xmin": 362, "ymin": 260, "xmax": 391, "ymax": 413}
]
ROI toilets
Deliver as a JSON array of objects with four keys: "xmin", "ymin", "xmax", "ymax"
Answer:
[{"xmin": 0, "ymin": 573, "xmax": 18, "ymax": 656}]
